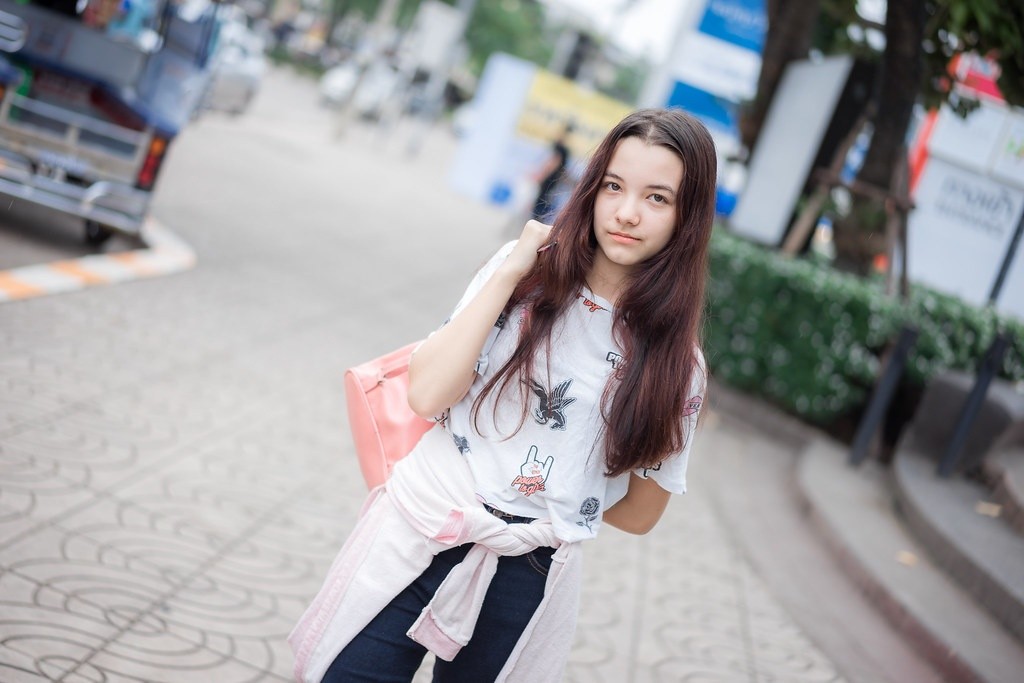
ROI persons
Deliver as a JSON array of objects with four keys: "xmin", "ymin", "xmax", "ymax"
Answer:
[
  {"xmin": 288, "ymin": 109, "xmax": 717, "ymax": 683},
  {"xmin": 527, "ymin": 122, "xmax": 577, "ymax": 224}
]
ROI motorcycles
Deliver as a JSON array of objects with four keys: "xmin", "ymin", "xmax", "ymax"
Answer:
[{"xmin": 0, "ymin": 0, "xmax": 218, "ymax": 246}]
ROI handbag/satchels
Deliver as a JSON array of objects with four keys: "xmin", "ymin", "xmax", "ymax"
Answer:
[{"xmin": 344, "ymin": 340, "xmax": 440, "ymax": 491}]
